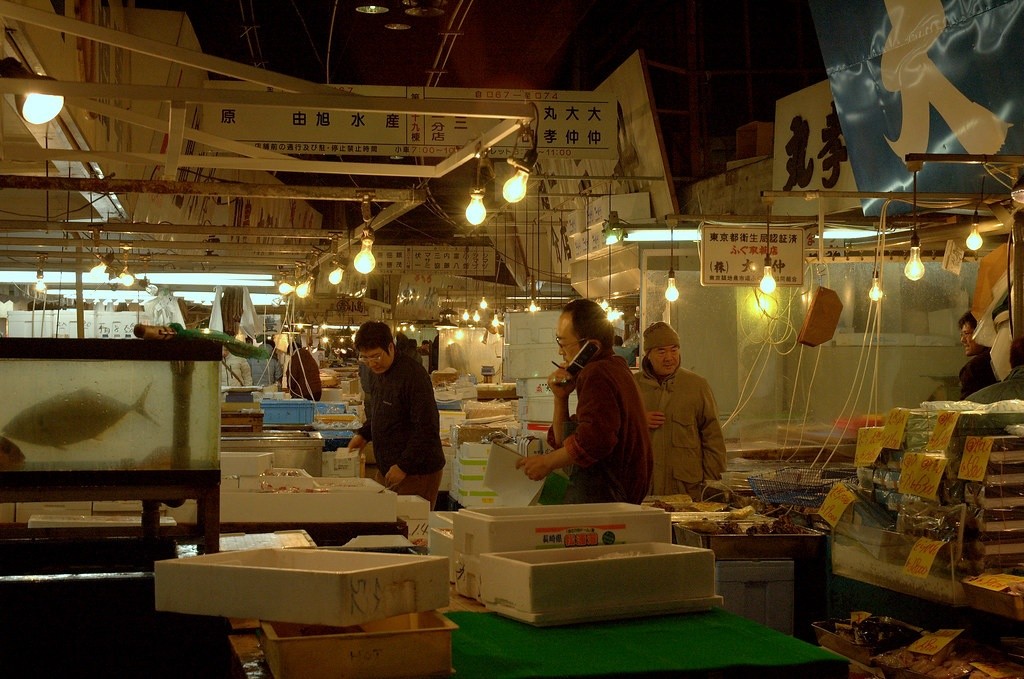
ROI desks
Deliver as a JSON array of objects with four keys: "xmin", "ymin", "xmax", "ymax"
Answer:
[{"xmin": 228, "ymin": 606, "xmax": 849, "ymax": 679}]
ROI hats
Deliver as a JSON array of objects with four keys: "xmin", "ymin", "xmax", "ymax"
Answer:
[{"xmin": 643, "ymin": 320, "xmax": 680, "ymax": 351}]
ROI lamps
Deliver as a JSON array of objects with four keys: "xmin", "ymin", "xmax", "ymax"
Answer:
[
  {"xmin": 0, "ymin": 55, "xmax": 64, "ymax": 125},
  {"xmin": 432, "ymin": 292, "xmax": 459, "ymax": 330}
]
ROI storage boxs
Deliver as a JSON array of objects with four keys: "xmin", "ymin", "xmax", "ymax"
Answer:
[{"xmin": 0, "ymin": 309, "xmax": 1023, "ymax": 679}]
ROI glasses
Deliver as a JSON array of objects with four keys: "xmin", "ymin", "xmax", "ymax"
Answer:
[
  {"xmin": 556, "ymin": 334, "xmax": 593, "ymax": 348},
  {"xmin": 358, "ymin": 345, "xmax": 384, "ymax": 363}
]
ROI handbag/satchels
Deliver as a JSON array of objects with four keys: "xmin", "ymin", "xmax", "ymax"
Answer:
[{"xmin": 239, "ymin": 286, "xmax": 264, "ymax": 339}]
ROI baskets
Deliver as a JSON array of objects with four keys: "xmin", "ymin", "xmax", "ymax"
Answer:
[{"xmin": 747, "ymin": 466, "xmax": 859, "ymax": 508}]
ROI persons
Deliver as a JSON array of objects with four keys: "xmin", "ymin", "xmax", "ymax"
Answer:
[
  {"xmin": 287, "ymin": 342, "xmax": 323, "ymax": 403},
  {"xmin": 515, "ymin": 299, "xmax": 651, "ymax": 504},
  {"xmin": 344, "ymin": 322, "xmax": 445, "ymax": 512},
  {"xmin": 224, "ymin": 338, "xmax": 281, "ymax": 389},
  {"xmin": 634, "ymin": 322, "xmax": 727, "ymax": 501},
  {"xmin": 957, "ymin": 308, "xmax": 1024, "ymax": 403},
  {"xmin": 397, "ymin": 332, "xmax": 440, "ymax": 373},
  {"xmin": 612, "ymin": 335, "xmax": 634, "ymax": 365}
]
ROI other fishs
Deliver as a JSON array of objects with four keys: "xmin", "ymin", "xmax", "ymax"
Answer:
[{"xmin": 0, "ymin": 380, "xmax": 162, "ymax": 451}]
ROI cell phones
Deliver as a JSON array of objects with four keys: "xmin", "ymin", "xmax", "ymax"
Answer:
[{"xmin": 565, "ymin": 340, "xmax": 600, "ymax": 380}]
